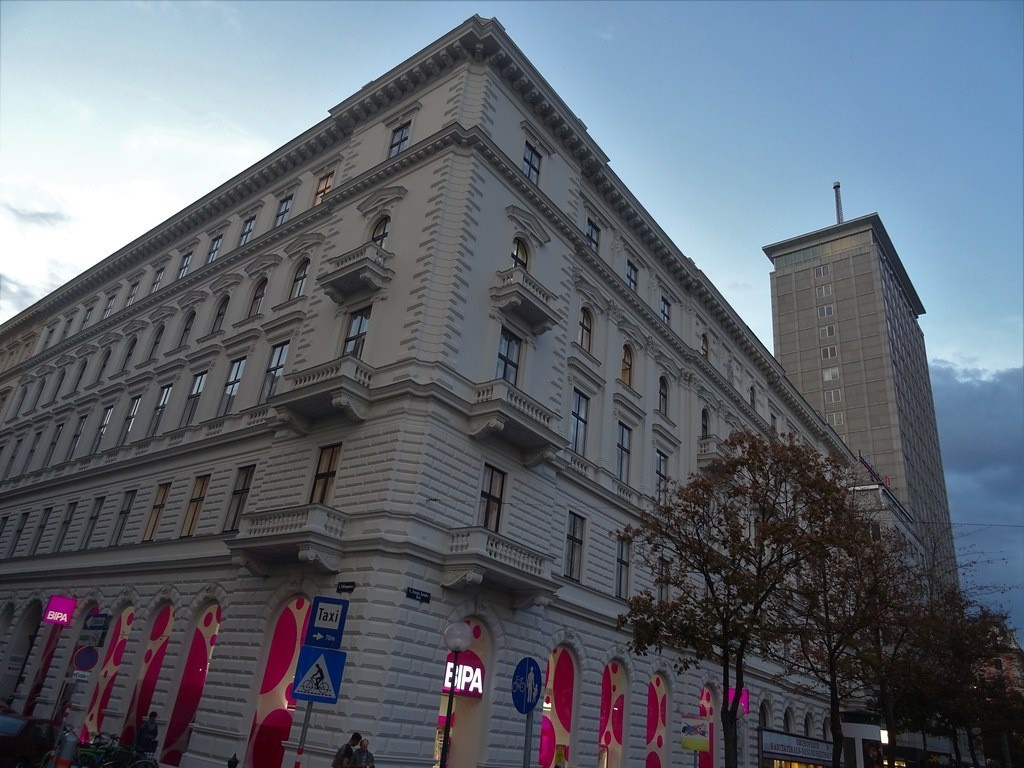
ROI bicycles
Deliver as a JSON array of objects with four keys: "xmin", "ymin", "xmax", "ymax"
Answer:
[{"xmin": 40, "ymin": 731, "xmax": 159, "ymax": 768}]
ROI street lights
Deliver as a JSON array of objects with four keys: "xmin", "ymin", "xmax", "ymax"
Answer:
[{"xmin": 439, "ymin": 622, "xmax": 475, "ymax": 768}]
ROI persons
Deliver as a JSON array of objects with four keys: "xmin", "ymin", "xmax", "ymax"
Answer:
[
  {"xmin": 354, "ymin": 739, "xmax": 374, "ymax": 768},
  {"xmin": 332, "ymin": 732, "xmax": 362, "ymax": 768},
  {"xmin": 135, "ymin": 711, "xmax": 158, "ymax": 751}
]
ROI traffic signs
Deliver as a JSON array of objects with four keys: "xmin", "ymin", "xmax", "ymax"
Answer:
[
  {"xmin": 304, "ymin": 595, "xmax": 350, "ymax": 650},
  {"xmin": 78, "ymin": 614, "xmax": 108, "ymax": 647}
]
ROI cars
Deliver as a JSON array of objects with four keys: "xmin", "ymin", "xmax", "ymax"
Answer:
[{"xmin": 0, "ymin": 714, "xmax": 45, "ymax": 768}]
